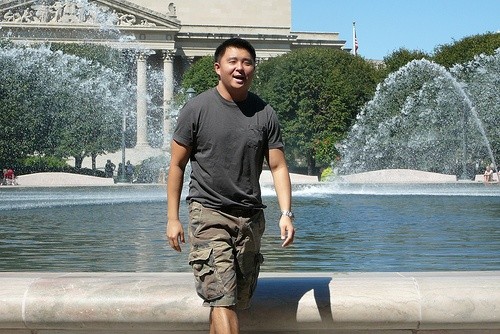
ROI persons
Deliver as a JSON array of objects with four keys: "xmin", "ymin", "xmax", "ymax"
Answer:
[
  {"xmin": 0, "ymin": 167, "xmax": 15, "ymax": 185},
  {"xmin": 168, "ymin": 38, "xmax": 296, "ymax": 334},
  {"xmin": 104, "ymin": 158, "xmax": 135, "ymax": 184},
  {"xmin": 484, "ymin": 166, "xmax": 493, "ymax": 183},
  {"xmin": 3, "ymin": 2, "xmax": 136, "ymax": 24}
]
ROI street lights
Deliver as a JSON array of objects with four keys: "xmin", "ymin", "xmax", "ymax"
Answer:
[
  {"xmin": 117, "ymin": 87, "xmax": 126, "ymax": 182},
  {"xmin": 460, "ymin": 82, "xmax": 469, "ymax": 176}
]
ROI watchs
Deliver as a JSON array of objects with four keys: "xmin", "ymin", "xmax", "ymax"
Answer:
[{"xmin": 279, "ymin": 211, "xmax": 293, "ymax": 218}]
